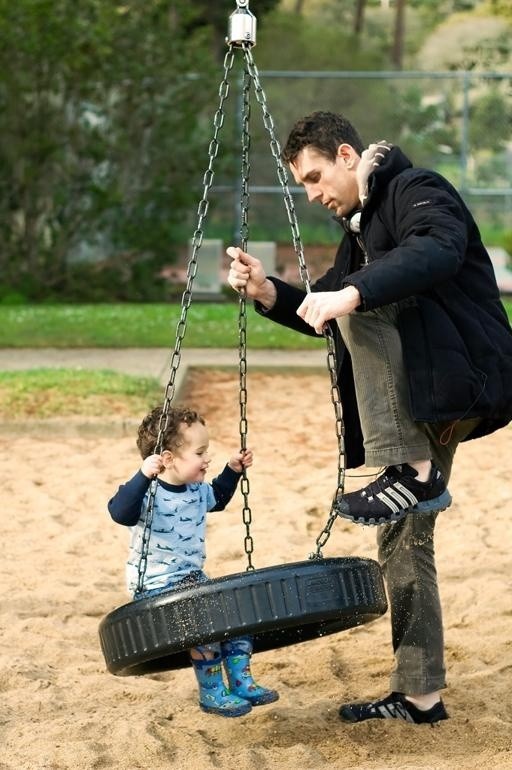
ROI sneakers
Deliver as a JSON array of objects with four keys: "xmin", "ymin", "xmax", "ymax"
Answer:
[
  {"xmin": 337, "ymin": 688, "xmax": 452, "ymax": 729},
  {"xmin": 332, "ymin": 459, "xmax": 454, "ymax": 527}
]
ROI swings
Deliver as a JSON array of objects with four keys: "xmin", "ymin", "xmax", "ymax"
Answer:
[{"xmin": 99, "ymin": 0, "xmax": 387, "ymax": 676}]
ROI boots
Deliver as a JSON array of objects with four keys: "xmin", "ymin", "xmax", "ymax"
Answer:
[
  {"xmin": 220, "ymin": 634, "xmax": 281, "ymax": 708},
  {"xmin": 190, "ymin": 655, "xmax": 253, "ymax": 719}
]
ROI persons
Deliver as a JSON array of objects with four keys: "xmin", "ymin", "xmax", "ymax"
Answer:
[
  {"xmin": 226, "ymin": 111, "xmax": 512, "ymax": 725},
  {"xmin": 108, "ymin": 405, "xmax": 279, "ymax": 717}
]
